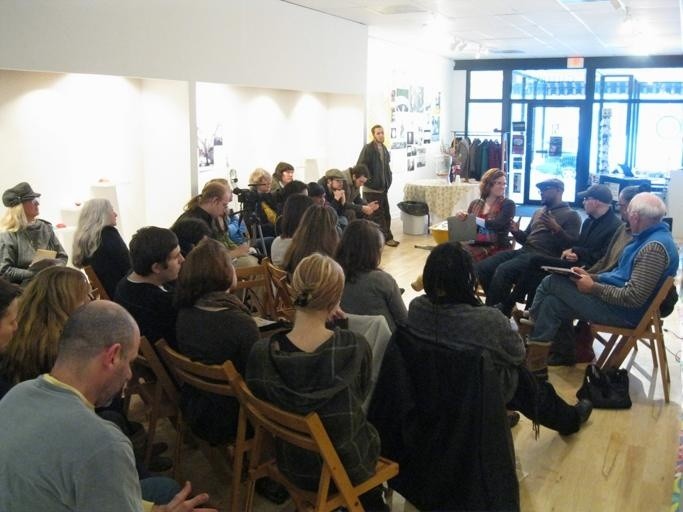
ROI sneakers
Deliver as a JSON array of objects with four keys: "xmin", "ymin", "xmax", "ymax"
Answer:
[{"xmin": 411, "ymin": 278, "xmax": 423, "ymax": 291}]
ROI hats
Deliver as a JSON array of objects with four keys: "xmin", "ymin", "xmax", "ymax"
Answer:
[
  {"xmin": 536, "ymin": 179, "xmax": 563, "ymax": 191},
  {"xmin": 578, "ymin": 184, "xmax": 613, "ymax": 204},
  {"xmin": 325, "ymin": 169, "xmax": 347, "ymax": 182},
  {"xmin": 4, "ymin": 182, "xmax": 42, "ymax": 209}
]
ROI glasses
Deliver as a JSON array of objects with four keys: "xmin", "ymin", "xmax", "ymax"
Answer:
[
  {"xmin": 538, "ymin": 186, "xmax": 554, "ymax": 191},
  {"xmin": 583, "ymin": 196, "xmax": 596, "ymax": 200},
  {"xmin": 492, "ymin": 181, "xmax": 507, "ymax": 187},
  {"xmin": 615, "ymin": 202, "xmax": 624, "ymax": 207}
]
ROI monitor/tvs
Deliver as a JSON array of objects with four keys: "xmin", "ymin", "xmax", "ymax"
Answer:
[{"xmin": 618, "ymin": 163, "xmax": 634, "ymax": 177}]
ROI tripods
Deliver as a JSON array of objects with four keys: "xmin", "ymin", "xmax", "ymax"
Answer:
[{"xmin": 248, "ymin": 209, "xmax": 268, "ymax": 258}]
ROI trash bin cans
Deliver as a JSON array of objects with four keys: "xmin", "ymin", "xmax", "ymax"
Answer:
[{"xmin": 397, "ymin": 201, "xmax": 429, "ymax": 235}]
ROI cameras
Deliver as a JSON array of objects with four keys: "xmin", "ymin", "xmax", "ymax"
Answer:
[{"xmin": 233, "ymin": 184, "xmax": 274, "ymax": 208}]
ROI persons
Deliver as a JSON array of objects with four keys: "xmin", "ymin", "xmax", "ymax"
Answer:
[
  {"xmin": 327, "ymin": 221, "xmax": 415, "ymax": 337},
  {"xmin": 0, "ymin": 179, "xmax": 67, "ymax": 294},
  {"xmin": 478, "ymin": 178, "xmax": 679, "ymax": 371},
  {"xmin": 73, "ymin": 196, "xmax": 130, "ymax": 296},
  {"xmin": 354, "ymin": 123, "xmax": 401, "ymax": 247},
  {"xmin": 279, "ymin": 201, "xmax": 345, "ymax": 283},
  {"xmin": 115, "ymin": 220, "xmax": 185, "ymax": 335},
  {"xmin": 411, "ymin": 166, "xmax": 516, "ymax": 293},
  {"xmin": 0, "ymin": 297, "xmax": 216, "ymax": 511},
  {"xmin": 371, "ymin": 242, "xmax": 594, "ymax": 511},
  {"xmin": 245, "ymin": 251, "xmax": 380, "ymax": 493},
  {"xmin": 169, "ymin": 161, "xmax": 390, "ymax": 280},
  {"xmin": 0, "ymin": 266, "xmax": 102, "ymax": 388},
  {"xmin": 168, "ymin": 237, "xmax": 261, "ymax": 435}
]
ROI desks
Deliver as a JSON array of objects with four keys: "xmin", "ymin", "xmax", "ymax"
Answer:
[{"xmin": 402, "ymin": 177, "xmax": 483, "ymax": 230}]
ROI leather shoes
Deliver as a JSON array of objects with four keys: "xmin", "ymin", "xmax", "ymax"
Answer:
[
  {"xmin": 559, "ymin": 399, "xmax": 593, "ymax": 436},
  {"xmin": 148, "ymin": 455, "xmax": 173, "ymax": 473},
  {"xmin": 151, "ymin": 442, "xmax": 168, "ymax": 455},
  {"xmin": 386, "ymin": 240, "xmax": 399, "ymax": 247}
]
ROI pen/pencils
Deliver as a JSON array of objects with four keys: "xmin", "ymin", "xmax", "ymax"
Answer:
[{"xmin": 580, "ymin": 265, "xmax": 586, "ymax": 268}]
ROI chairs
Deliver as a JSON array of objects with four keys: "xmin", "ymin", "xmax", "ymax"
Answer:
[
  {"xmin": 79, "ymin": 264, "xmax": 112, "ymax": 305},
  {"xmin": 585, "ymin": 275, "xmax": 678, "ymax": 407},
  {"xmin": 232, "ymin": 254, "xmax": 295, "ymax": 324}
]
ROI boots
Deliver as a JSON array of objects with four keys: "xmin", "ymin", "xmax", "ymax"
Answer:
[
  {"xmin": 524, "ymin": 342, "xmax": 550, "ymax": 380},
  {"xmin": 518, "ymin": 318, "xmax": 534, "ymax": 340}
]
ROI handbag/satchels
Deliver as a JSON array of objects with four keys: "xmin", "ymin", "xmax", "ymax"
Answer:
[
  {"xmin": 575, "ymin": 365, "xmax": 632, "ymax": 410},
  {"xmin": 568, "ymin": 320, "xmax": 596, "ymax": 364}
]
